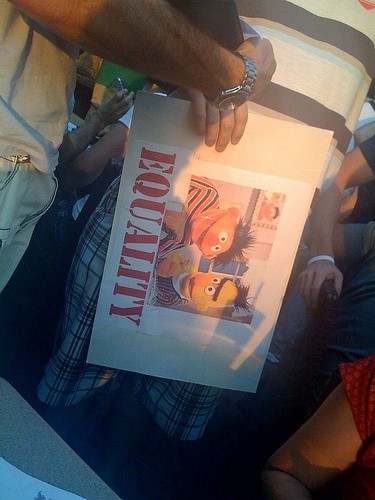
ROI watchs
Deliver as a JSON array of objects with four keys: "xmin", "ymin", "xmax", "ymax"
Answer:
[{"xmin": 203, "ymin": 51, "xmax": 258, "ymax": 114}]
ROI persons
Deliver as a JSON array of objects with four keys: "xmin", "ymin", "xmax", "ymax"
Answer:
[
  {"xmin": 338, "ymin": 95, "xmax": 375, "ymax": 214},
  {"xmin": 53, "ymin": 46, "xmax": 161, "ymax": 255},
  {"xmin": 264, "ymin": 351, "xmax": 374, "ymax": 500},
  {"xmin": 300, "ymin": 202, "xmax": 374, "ymax": 411},
  {"xmin": 300, "ymin": 135, "xmax": 375, "ymax": 309},
  {"xmin": 32, "ymin": 0, "xmax": 375, "ymax": 500},
  {"xmin": 0, "ymin": 0, "xmax": 276, "ymax": 349}
]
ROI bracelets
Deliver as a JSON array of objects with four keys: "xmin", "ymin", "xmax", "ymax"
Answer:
[{"xmin": 305, "ymin": 255, "xmax": 335, "ymax": 265}]
